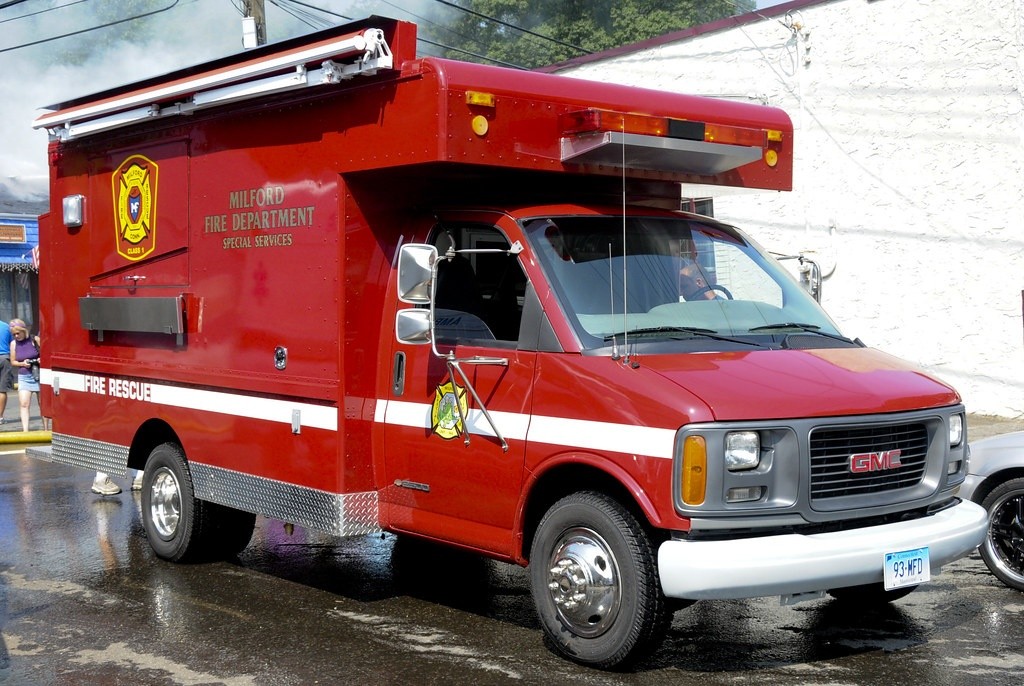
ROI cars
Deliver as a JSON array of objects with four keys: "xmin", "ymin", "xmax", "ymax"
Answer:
[{"xmin": 953, "ymin": 430, "xmax": 1024, "ymax": 593}]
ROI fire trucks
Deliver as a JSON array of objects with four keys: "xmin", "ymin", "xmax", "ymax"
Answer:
[{"xmin": 36, "ymin": 11, "xmax": 996, "ymax": 672}]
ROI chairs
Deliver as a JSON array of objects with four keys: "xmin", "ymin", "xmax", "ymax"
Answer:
[{"xmin": 432, "ymin": 253, "xmax": 487, "ymax": 324}]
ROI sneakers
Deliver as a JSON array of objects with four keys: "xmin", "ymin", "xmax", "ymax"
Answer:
[
  {"xmin": 131, "ymin": 478, "xmax": 143, "ymax": 491},
  {"xmin": 91, "ymin": 477, "xmax": 121, "ymax": 495}
]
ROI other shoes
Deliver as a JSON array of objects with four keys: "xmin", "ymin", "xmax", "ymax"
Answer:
[{"xmin": 0, "ymin": 417, "xmax": 7, "ymax": 424}]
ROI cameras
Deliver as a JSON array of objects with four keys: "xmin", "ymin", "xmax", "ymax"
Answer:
[{"xmin": 28, "ymin": 359, "xmax": 40, "ymax": 382}]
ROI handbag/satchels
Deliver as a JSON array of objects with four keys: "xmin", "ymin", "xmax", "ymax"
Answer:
[{"xmin": 31, "ymin": 363, "xmax": 39, "ymax": 382}]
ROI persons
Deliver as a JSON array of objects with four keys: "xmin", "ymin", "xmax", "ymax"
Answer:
[
  {"xmin": 91, "ymin": 469, "xmax": 146, "ymax": 496},
  {"xmin": 9, "ymin": 319, "xmax": 49, "ymax": 431},
  {"xmin": 92, "ymin": 497, "xmax": 123, "ymax": 569},
  {"xmin": 0, "ymin": 320, "xmax": 14, "ymax": 425},
  {"xmin": 680, "ymin": 264, "xmax": 726, "ymax": 300}
]
